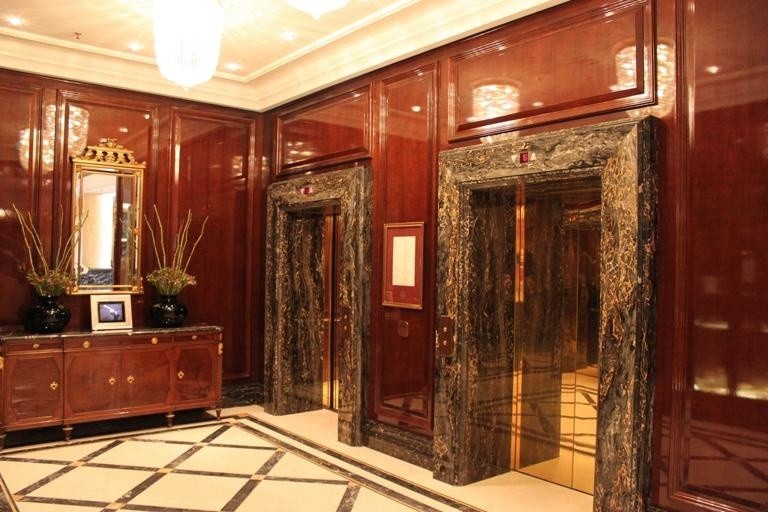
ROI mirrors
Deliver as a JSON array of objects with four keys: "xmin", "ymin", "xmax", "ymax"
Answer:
[{"xmin": 66, "ymin": 137, "xmax": 146, "ymax": 297}]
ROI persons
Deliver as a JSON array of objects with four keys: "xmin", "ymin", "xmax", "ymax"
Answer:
[{"xmin": 111, "ymin": 309, "xmax": 120, "ymax": 320}]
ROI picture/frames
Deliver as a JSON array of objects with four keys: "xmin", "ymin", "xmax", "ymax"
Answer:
[
  {"xmin": 378, "ymin": 221, "xmax": 427, "ymax": 313},
  {"xmin": 90, "ymin": 294, "xmax": 134, "ymax": 332}
]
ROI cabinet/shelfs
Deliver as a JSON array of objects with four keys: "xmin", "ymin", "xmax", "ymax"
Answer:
[
  {"xmin": 64, "ymin": 331, "xmax": 171, "ymax": 426},
  {"xmin": 171, "ymin": 328, "xmax": 222, "ymax": 414},
  {"xmin": 1, "ymin": 326, "xmax": 63, "ymax": 433}
]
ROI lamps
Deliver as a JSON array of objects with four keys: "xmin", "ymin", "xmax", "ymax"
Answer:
[
  {"xmin": 469, "ymin": 76, "xmax": 522, "ymax": 144},
  {"xmin": 610, "ymin": 36, "xmax": 677, "ymax": 123}
]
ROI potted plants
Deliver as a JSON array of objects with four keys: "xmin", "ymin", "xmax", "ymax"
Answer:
[
  {"xmin": 137, "ymin": 204, "xmax": 211, "ymax": 326},
  {"xmin": 7, "ymin": 202, "xmax": 90, "ymax": 335}
]
